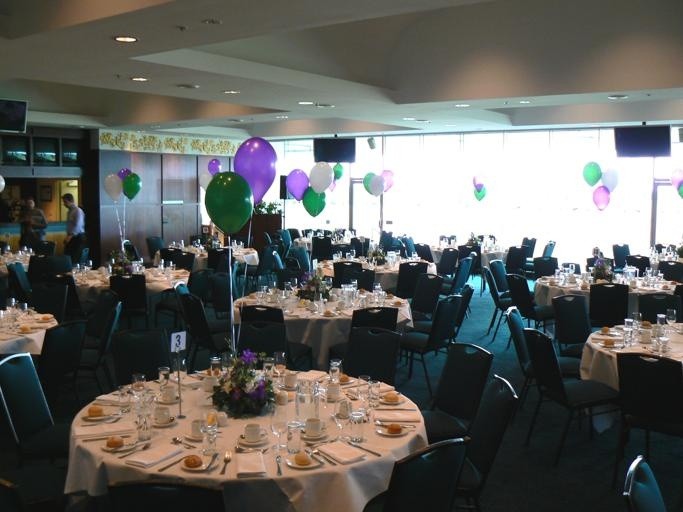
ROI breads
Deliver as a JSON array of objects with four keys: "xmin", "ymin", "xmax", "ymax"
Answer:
[
  {"xmin": 295, "ymin": 453, "xmax": 309, "ymax": 465},
  {"xmin": 208, "ymin": 368, "xmax": 220, "ymax": 376},
  {"xmin": 605, "ymin": 339, "xmax": 615, "ymax": 345},
  {"xmin": 21, "ymin": 326, "xmax": 31, "ymax": 333},
  {"xmin": 387, "ymin": 424, "xmax": 402, "ymax": 434},
  {"xmin": 384, "ymin": 392, "xmax": 399, "ymax": 402},
  {"xmin": 88, "ymin": 406, "xmax": 103, "ymax": 416},
  {"xmin": 601, "ymin": 327, "xmax": 609, "ymax": 334},
  {"xmin": 642, "ymin": 321, "xmax": 650, "ymax": 326},
  {"xmin": 42, "ymin": 314, "xmax": 53, "ymax": 320},
  {"xmin": 339, "ymin": 374, "xmax": 349, "ymax": 382},
  {"xmin": 184, "ymin": 456, "xmax": 202, "ymax": 469},
  {"xmin": 106, "ymin": 437, "xmax": 124, "ymax": 448}
]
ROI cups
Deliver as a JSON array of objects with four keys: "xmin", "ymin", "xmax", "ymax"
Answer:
[
  {"xmin": 256, "ymin": 230, "xmax": 421, "ymax": 313},
  {"xmin": 554, "ymin": 245, "xmax": 680, "ymax": 347},
  {"xmin": 245, "ymin": 424, "xmax": 268, "ymax": 442},
  {"xmin": 1, "ymin": 239, "xmax": 245, "ymax": 316},
  {"xmin": 131, "ymin": 352, "xmax": 233, "ymax": 455},
  {"xmin": 263, "ymin": 352, "xmax": 380, "ymax": 453}
]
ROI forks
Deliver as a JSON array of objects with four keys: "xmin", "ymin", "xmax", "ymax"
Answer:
[{"xmin": 220, "ymin": 448, "xmax": 232, "ymax": 474}]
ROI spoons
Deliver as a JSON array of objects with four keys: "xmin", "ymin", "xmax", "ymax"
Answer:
[{"xmin": 276, "ymin": 455, "xmax": 282, "ymax": 476}]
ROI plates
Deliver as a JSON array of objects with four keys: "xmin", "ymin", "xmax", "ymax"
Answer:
[
  {"xmin": 179, "ymin": 455, "xmax": 219, "ymax": 470},
  {"xmin": 81, "ymin": 415, "xmax": 112, "ymax": 421},
  {"xmin": 379, "ymin": 399, "xmax": 406, "ymax": 405},
  {"xmin": 239, "ymin": 438, "xmax": 269, "ymax": 446},
  {"xmin": 101, "ymin": 442, "xmax": 136, "ymax": 452},
  {"xmin": 285, "ymin": 455, "xmax": 320, "ymax": 468},
  {"xmin": 377, "ymin": 428, "xmax": 408, "ymax": 436}
]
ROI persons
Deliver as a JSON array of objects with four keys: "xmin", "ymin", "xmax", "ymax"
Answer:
[
  {"xmin": 62, "ymin": 193, "xmax": 86, "ymax": 265},
  {"xmin": 18, "ymin": 197, "xmax": 48, "ymax": 250}
]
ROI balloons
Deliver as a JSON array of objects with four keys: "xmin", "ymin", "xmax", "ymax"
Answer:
[
  {"xmin": 583, "ymin": 161, "xmax": 618, "ymax": 212},
  {"xmin": 363, "ymin": 170, "xmax": 395, "ymax": 198},
  {"xmin": 208, "ymin": 159, "xmax": 221, "ymax": 176},
  {"xmin": 105, "ymin": 168, "xmax": 142, "ymax": 204},
  {"xmin": 205, "ymin": 172, "xmax": 254, "ymax": 235},
  {"xmin": 233, "ymin": 137, "xmax": 278, "ymax": 205},
  {"xmin": 473, "ymin": 177, "xmax": 483, "ymax": 193},
  {"xmin": 475, "ymin": 187, "xmax": 486, "ymax": 201},
  {"xmin": 286, "ymin": 161, "xmax": 343, "ymax": 218},
  {"xmin": 0, "ymin": 175, "xmax": 6, "ymax": 193},
  {"xmin": 674, "ymin": 172, "xmax": 683, "ymax": 197}
]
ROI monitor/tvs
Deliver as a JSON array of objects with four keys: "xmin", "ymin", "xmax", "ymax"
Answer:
[{"xmin": 0, "ymin": 98, "xmax": 29, "ymax": 134}]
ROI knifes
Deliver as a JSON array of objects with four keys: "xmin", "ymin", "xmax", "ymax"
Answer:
[
  {"xmin": 376, "ymin": 407, "xmax": 420, "ymax": 412},
  {"xmin": 83, "ymin": 435, "xmax": 129, "ymax": 442},
  {"xmin": 383, "ymin": 419, "xmax": 420, "ymax": 424},
  {"xmin": 158, "ymin": 457, "xmax": 186, "ymax": 472},
  {"xmin": 347, "ymin": 441, "xmax": 382, "ymax": 457}
]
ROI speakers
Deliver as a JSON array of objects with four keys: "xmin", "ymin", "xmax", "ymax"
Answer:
[
  {"xmin": 614, "ymin": 125, "xmax": 672, "ymax": 157},
  {"xmin": 280, "ymin": 176, "xmax": 294, "ymax": 200},
  {"xmin": 313, "ymin": 138, "xmax": 356, "ymax": 163}
]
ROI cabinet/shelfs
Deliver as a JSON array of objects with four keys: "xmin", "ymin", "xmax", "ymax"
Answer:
[{"xmin": 224, "ymin": 211, "xmax": 282, "ymax": 249}]
